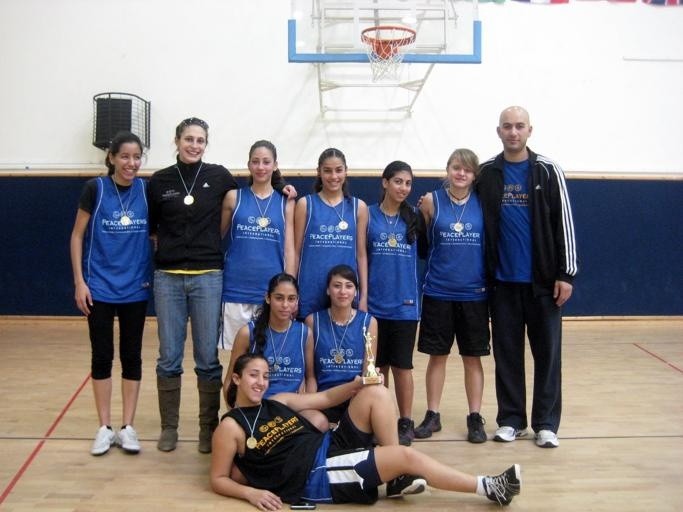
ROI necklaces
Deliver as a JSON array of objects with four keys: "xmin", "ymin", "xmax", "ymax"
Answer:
[{"xmin": 448, "ymin": 189, "xmax": 469, "ymax": 204}]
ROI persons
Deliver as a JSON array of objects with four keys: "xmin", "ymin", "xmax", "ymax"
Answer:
[
  {"xmin": 416, "ymin": 105, "xmax": 577, "ymax": 448},
  {"xmin": 366, "ymin": 161, "xmax": 430, "ymax": 446},
  {"xmin": 223, "ymin": 273, "xmax": 317, "ymax": 413},
  {"xmin": 412, "ymin": 148, "xmax": 491, "ymax": 443},
  {"xmin": 71, "ymin": 131, "xmax": 158, "ymax": 455},
  {"xmin": 298, "ymin": 264, "xmax": 378, "ymax": 394},
  {"xmin": 154, "ymin": 140, "xmax": 299, "ymax": 350},
  {"xmin": 295, "ymin": 147, "xmax": 368, "ymax": 321},
  {"xmin": 145, "ymin": 117, "xmax": 297, "ymax": 454},
  {"xmin": 209, "ymin": 352, "xmax": 522, "ymax": 512}
]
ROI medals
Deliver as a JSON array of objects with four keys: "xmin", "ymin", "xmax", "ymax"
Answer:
[
  {"xmin": 328, "ymin": 307, "xmax": 353, "ymax": 364},
  {"xmin": 447, "ymin": 187, "xmax": 469, "ymax": 232},
  {"xmin": 174, "ymin": 162, "xmax": 203, "ymax": 205},
  {"xmin": 322, "ymin": 189, "xmax": 348, "ymax": 230},
  {"xmin": 268, "ymin": 320, "xmax": 292, "ymax": 371},
  {"xmin": 234, "ymin": 400, "xmax": 262, "ymax": 450},
  {"xmin": 112, "ymin": 174, "xmax": 134, "ymax": 226},
  {"xmin": 251, "ymin": 185, "xmax": 273, "ymax": 227},
  {"xmin": 382, "ymin": 202, "xmax": 400, "ymax": 248}
]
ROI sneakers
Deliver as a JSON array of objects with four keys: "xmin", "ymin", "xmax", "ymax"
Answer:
[
  {"xmin": 467, "ymin": 414, "xmax": 488, "ymax": 443},
  {"xmin": 90, "ymin": 425, "xmax": 117, "ymax": 455},
  {"xmin": 533, "ymin": 430, "xmax": 559, "ymax": 448},
  {"xmin": 385, "ymin": 475, "xmax": 427, "ymax": 499},
  {"xmin": 115, "ymin": 424, "xmax": 140, "ymax": 453},
  {"xmin": 414, "ymin": 410, "xmax": 441, "ymax": 439},
  {"xmin": 493, "ymin": 425, "xmax": 528, "ymax": 442},
  {"xmin": 482, "ymin": 464, "xmax": 522, "ymax": 507},
  {"xmin": 398, "ymin": 417, "xmax": 415, "ymax": 446}
]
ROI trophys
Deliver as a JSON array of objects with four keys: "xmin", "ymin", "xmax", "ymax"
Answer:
[{"xmin": 361, "ymin": 313, "xmax": 381, "ymax": 385}]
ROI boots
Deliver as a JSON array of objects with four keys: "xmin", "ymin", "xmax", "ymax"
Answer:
[
  {"xmin": 156, "ymin": 375, "xmax": 182, "ymax": 452},
  {"xmin": 196, "ymin": 381, "xmax": 222, "ymax": 453}
]
